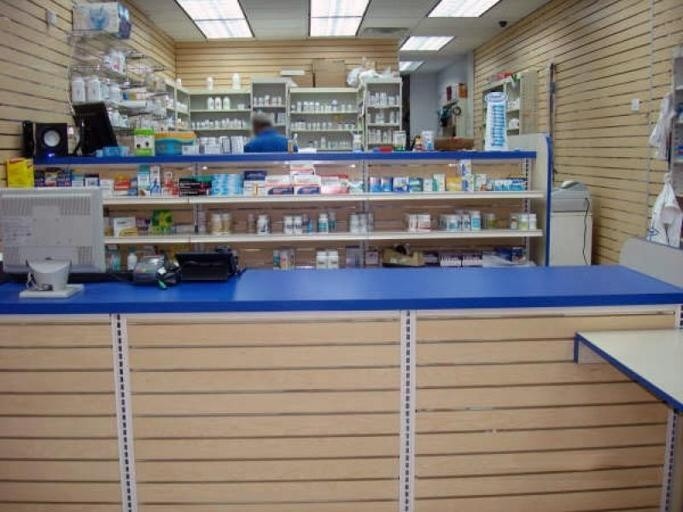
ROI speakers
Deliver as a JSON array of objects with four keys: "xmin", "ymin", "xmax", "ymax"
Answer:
[{"xmin": 24, "ymin": 120, "xmax": 67, "ymax": 158}]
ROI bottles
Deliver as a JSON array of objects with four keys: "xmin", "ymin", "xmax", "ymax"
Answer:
[
  {"xmin": 440, "ymin": 208, "xmax": 537, "ymax": 232},
  {"xmin": 245, "ymin": 213, "xmax": 271, "ymax": 236},
  {"xmin": 348, "ymin": 211, "xmax": 368, "ymax": 234},
  {"xmin": 317, "ymin": 212, "xmax": 336, "ymax": 234},
  {"xmin": 210, "ymin": 212, "xmax": 232, "ymax": 235},
  {"xmin": 124, "ymin": 247, "xmax": 137, "ymax": 271},
  {"xmin": 250, "ymin": 96, "xmax": 353, "ymax": 150},
  {"xmin": 271, "ymin": 246, "xmax": 295, "ymax": 271},
  {"xmin": 281, "ymin": 214, "xmax": 313, "ymax": 236},
  {"xmin": 175, "ymin": 70, "xmax": 247, "ymax": 144}
]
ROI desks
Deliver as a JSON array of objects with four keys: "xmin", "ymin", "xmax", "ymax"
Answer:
[{"xmin": 574, "ymin": 329, "xmax": 683, "ymax": 512}]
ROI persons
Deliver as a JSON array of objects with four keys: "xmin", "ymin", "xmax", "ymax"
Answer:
[{"xmin": 244, "ymin": 111, "xmax": 298, "ymax": 153}]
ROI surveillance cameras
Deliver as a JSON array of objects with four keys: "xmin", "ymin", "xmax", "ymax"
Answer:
[{"xmin": 498, "ymin": 21, "xmax": 508, "ymax": 28}]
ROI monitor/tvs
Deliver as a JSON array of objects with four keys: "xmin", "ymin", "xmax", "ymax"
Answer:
[
  {"xmin": 0, "ymin": 187, "xmax": 106, "ymax": 299},
  {"xmin": 70, "ymin": 101, "xmax": 119, "ymax": 158}
]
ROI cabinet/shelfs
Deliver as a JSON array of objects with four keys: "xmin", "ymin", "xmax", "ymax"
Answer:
[
  {"xmin": 670, "ymin": 49, "xmax": 683, "ymax": 242},
  {"xmin": 34, "ymin": 28, "xmax": 552, "ymax": 152},
  {"xmin": 33, "ymin": 131, "xmax": 552, "ymax": 267}
]
